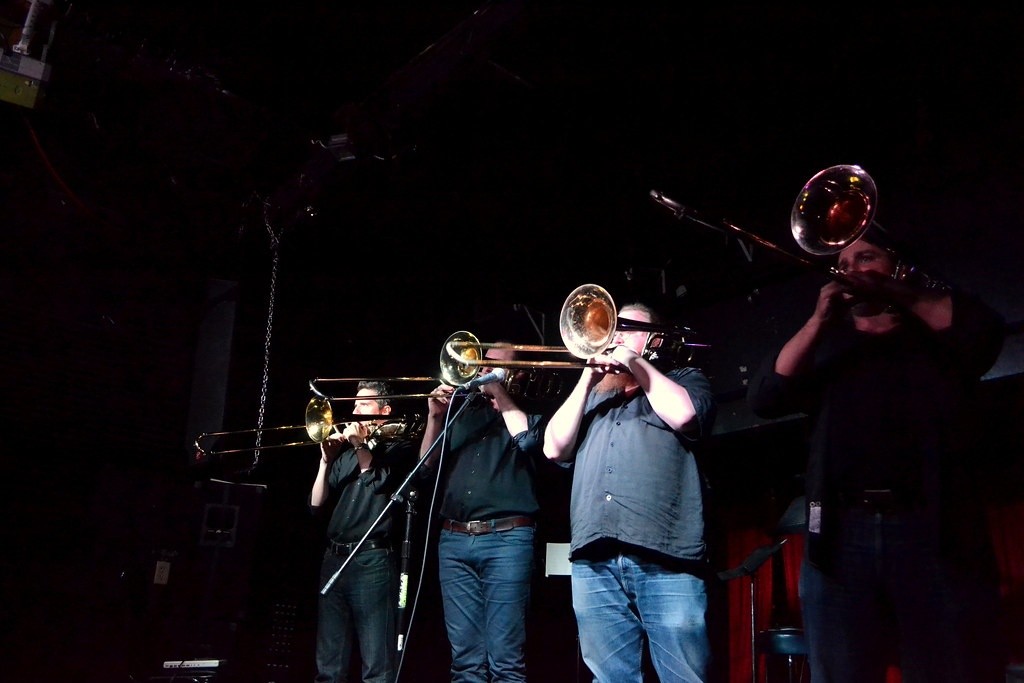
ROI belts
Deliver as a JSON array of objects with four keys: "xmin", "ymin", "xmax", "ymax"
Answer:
[
  {"xmin": 864, "ymin": 489, "xmax": 920, "ymax": 511},
  {"xmin": 327, "ymin": 538, "xmax": 389, "ymax": 556},
  {"xmin": 443, "ymin": 518, "xmax": 532, "ymax": 535}
]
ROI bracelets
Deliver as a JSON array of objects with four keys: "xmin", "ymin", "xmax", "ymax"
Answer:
[{"xmin": 355, "ymin": 442, "xmax": 367, "ymax": 452}]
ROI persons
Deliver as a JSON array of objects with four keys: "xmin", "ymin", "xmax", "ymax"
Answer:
[
  {"xmin": 542, "ymin": 299, "xmax": 715, "ymax": 683},
  {"xmin": 767, "ymin": 235, "xmax": 1007, "ymax": 683},
  {"xmin": 309, "ymin": 381, "xmax": 415, "ymax": 683},
  {"xmin": 418, "ymin": 341, "xmax": 559, "ymax": 683}
]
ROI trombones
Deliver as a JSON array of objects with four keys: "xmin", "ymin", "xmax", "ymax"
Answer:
[
  {"xmin": 445, "ymin": 283, "xmax": 712, "ymax": 371},
  {"xmin": 649, "ymin": 164, "xmax": 953, "ymax": 311},
  {"xmin": 195, "ymin": 395, "xmax": 428, "ymax": 456},
  {"xmin": 308, "ymin": 330, "xmax": 565, "ymax": 401}
]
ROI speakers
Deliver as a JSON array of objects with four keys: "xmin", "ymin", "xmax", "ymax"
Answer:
[{"xmin": 186, "ymin": 300, "xmax": 262, "ymax": 472}]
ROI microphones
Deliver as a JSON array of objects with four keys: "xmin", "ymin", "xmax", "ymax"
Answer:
[{"xmin": 457, "ymin": 367, "xmax": 506, "ymax": 392}]
